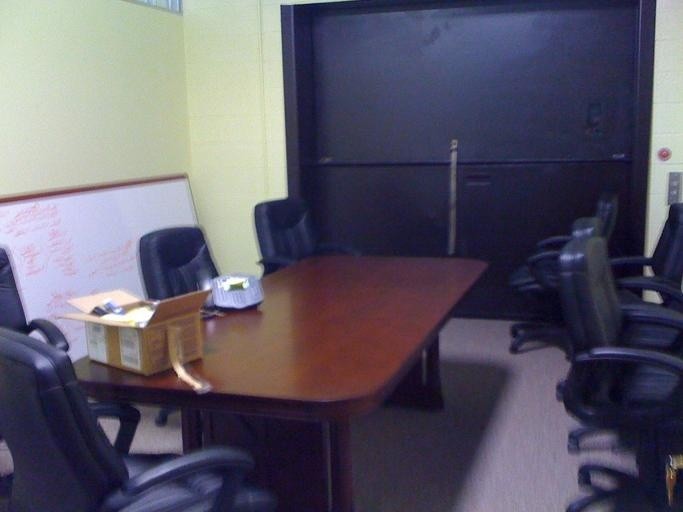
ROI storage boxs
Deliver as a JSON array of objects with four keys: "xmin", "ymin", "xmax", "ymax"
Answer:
[{"xmin": 59, "ymin": 290, "xmax": 213, "ymax": 377}]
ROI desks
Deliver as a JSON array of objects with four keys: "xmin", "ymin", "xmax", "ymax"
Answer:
[{"xmin": 73, "ymin": 255, "xmax": 490, "ymax": 512}]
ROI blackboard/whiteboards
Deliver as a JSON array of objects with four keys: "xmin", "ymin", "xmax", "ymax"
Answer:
[{"xmin": 0, "ymin": 174, "xmax": 199, "ymax": 477}]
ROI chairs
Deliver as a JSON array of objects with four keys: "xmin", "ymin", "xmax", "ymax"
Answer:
[
  {"xmin": 0, "ymin": 247, "xmax": 70, "ymax": 352},
  {"xmin": 139, "ymin": 227, "xmax": 263, "ymax": 440},
  {"xmin": 0, "ymin": 326, "xmax": 279, "ymax": 512},
  {"xmin": 553, "ymin": 241, "xmax": 682, "ymax": 510},
  {"xmin": 509, "ymin": 191, "xmax": 615, "ymax": 352},
  {"xmin": 253, "ymin": 199, "xmax": 362, "ymax": 278},
  {"xmin": 614, "ymin": 202, "xmax": 679, "ymax": 301}
]
